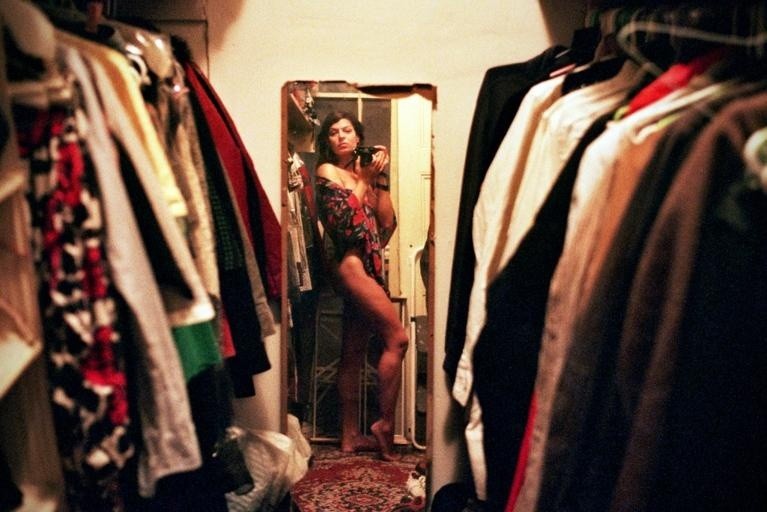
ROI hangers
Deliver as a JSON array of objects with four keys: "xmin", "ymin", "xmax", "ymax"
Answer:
[{"xmin": 576, "ymin": 2, "xmax": 764, "ymax": 187}]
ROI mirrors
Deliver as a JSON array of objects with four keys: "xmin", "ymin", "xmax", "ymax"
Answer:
[{"xmin": 271, "ymin": 78, "xmax": 441, "ymax": 512}]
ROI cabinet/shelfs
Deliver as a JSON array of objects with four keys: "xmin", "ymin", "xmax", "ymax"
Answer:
[{"xmin": 0, "ymin": 162, "xmax": 70, "ymax": 511}]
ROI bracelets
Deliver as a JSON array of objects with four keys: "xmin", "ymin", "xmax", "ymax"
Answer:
[
  {"xmin": 377, "ymin": 170, "xmax": 388, "ymax": 179},
  {"xmin": 375, "ymin": 182, "xmax": 389, "ymax": 191}
]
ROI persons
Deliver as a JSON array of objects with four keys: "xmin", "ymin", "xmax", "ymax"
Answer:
[{"xmin": 314, "ymin": 110, "xmax": 409, "ymax": 461}]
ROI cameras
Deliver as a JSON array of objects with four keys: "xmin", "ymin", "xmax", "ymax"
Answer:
[{"xmin": 355, "ymin": 147, "xmax": 374, "ymax": 163}]
